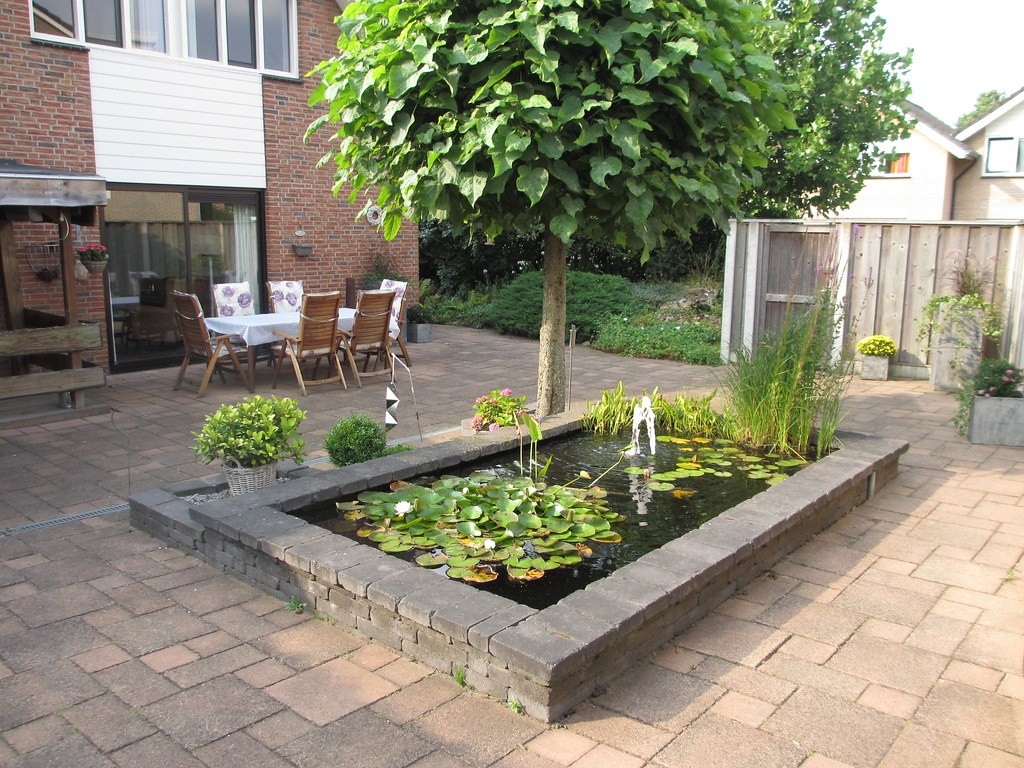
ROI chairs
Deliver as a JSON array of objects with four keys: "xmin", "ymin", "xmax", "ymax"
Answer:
[
  {"xmin": 326, "ymin": 290, "xmax": 396, "ymax": 388},
  {"xmin": 271, "ymin": 291, "xmax": 347, "ymax": 397},
  {"xmin": 362, "ymin": 278, "xmax": 413, "ymax": 372},
  {"xmin": 211, "ymin": 282, "xmax": 278, "ymax": 373},
  {"xmin": 267, "ymin": 279, "xmax": 305, "ymax": 369},
  {"xmin": 170, "ymin": 289, "xmax": 252, "ymax": 398},
  {"xmin": 114, "ymin": 274, "xmax": 232, "ymax": 351}
]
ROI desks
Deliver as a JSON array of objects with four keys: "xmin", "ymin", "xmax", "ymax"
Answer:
[
  {"xmin": 110, "ymin": 296, "xmax": 155, "ymax": 347},
  {"xmin": 204, "ymin": 307, "xmax": 400, "ymax": 393}
]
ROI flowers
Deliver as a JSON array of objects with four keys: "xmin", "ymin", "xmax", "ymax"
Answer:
[
  {"xmin": 949, "ymin": 358, "xmax": 1024, "ymax": 437},
  {"xmin": 472, "ymin": 388, "xmax": 544, "ymax": 432},
  {"xmin": 856, "ymin": 335, "xmax": 897, "ymax": 357},
  {"xmin": 77, "ymin": 244, "xmax": 109, "ymax": 261}
]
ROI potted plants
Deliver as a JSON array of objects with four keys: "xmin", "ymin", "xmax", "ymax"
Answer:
[
  {"xmin": 188, "ymin": 393, "xmax": 308, "ymax": 496},
  {"xmin": 405, "ymin": 302, "xmax": 434, "ymax": 343},
  {"xmin": 913, "ymin": 259, "xmax": 1004, "ymax": 394},
  {"xmin": 361, "ymin": 252, "xmax": 414, "ymax": 290}
]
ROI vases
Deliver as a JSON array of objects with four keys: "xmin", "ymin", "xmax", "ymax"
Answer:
[
  {"xmin": 81, "ymin": 261, "xmax": 108, "ymax": 275},
  {"xmin": 293, "ymin": 243, "xmax": 313, "ymax": 256},
  {"xmin": 860, "ymin": 355, "xmax": 889, "ymax": 382},
  {"xmin": 967, "ymin": 394, "xmax": 1024, "ymax": 446}
]
